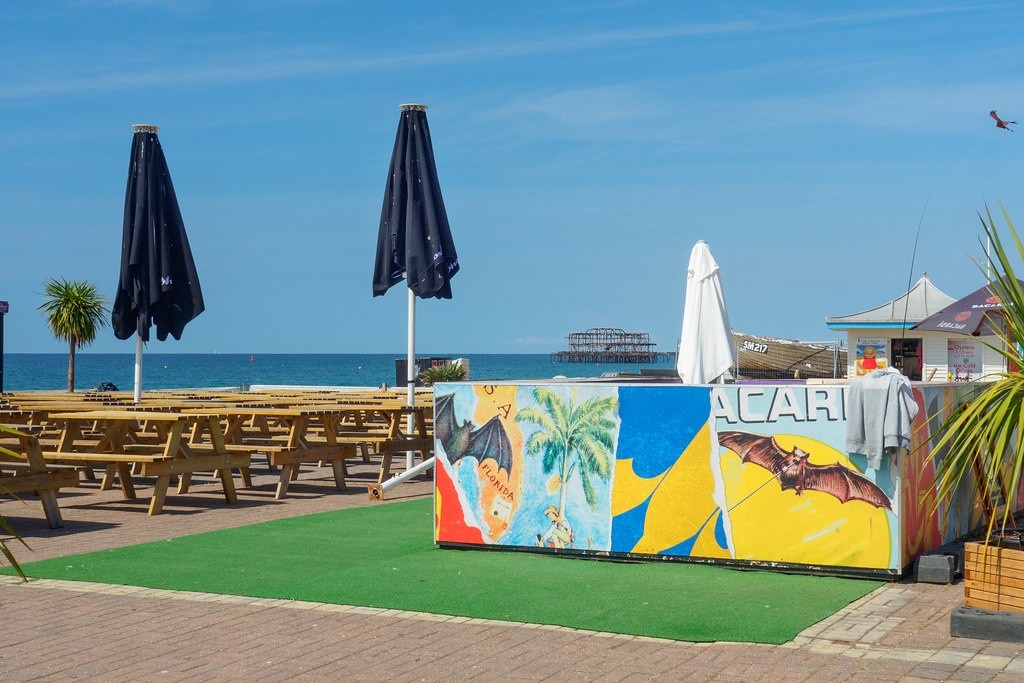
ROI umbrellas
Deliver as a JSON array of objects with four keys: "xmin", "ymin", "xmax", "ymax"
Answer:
[
  {"xmin": 909, "ymin": 272, "xmax": 1024, "ymax": 380},
  {"xmin": 371, "ymin": 104, "xmax": 460, "ymax": 471},
  {"xmin": 112, "ymin": 126, "xmax": 205, "ymax": 404},
  {"xmin": 675, "ymin": 239, "xmax": 738, "ymax": 386}
]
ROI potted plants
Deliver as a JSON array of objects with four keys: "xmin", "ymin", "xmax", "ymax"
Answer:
[{"xmin": 903, "ymin": 199, "xmax": 1024, "ymax": 615}]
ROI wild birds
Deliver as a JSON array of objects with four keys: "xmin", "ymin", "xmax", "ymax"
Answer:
[{"xmin": 990, "ymin": 109, "xmax": 1018, "ymax": 134}]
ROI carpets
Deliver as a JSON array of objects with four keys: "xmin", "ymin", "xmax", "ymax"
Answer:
[{"xmin": 1, "ymin": 494, "xmax": 888, "ymax": 646}]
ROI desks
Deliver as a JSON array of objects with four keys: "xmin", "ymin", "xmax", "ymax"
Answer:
[{"xmin": 5, "ymin": 389, "xmax": 434, "ymax": 531}]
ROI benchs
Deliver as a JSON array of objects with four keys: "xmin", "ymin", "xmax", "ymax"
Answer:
[{"xmin": 1, "ymin": 413, "xmax": 439, "ymax": 492}]
